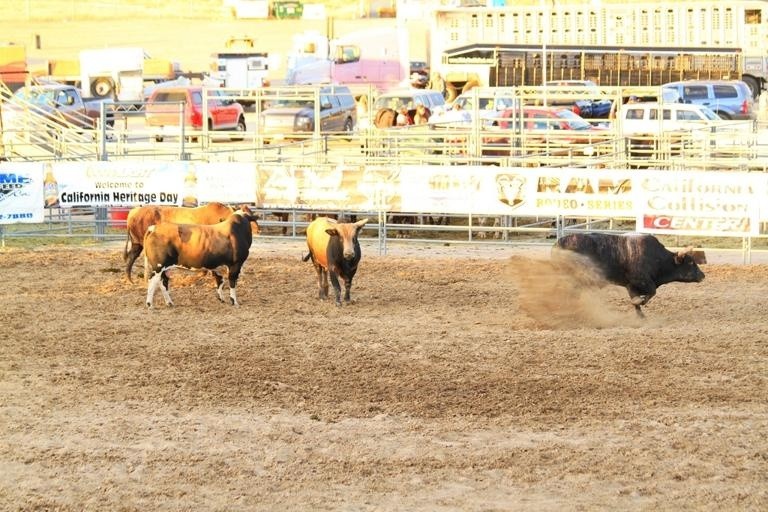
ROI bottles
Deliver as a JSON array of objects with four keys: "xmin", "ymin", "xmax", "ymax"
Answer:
[
  {"xmin": 182, "ymin": 161, "xmax": 199, "ymax": 208},
  {"xmin": 42, "ymin": 162, "xmax": 61, "ymax": 208}
]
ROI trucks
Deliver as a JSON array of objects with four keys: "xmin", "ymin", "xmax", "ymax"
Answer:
[{"xmin": 743, "ymin": 55, "xmax": 768, "ymax": 97}]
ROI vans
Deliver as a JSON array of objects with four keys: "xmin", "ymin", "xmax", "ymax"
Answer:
[
  {"xmin": 618, "ymin": 105, "xmax": 721, "ymax": 133},
  {"xmin": 658, "ymin": 79, "xmax": 752, "ymax": 120}
]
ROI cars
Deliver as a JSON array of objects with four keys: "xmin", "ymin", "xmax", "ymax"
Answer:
[
  {"xmin": 542, "ymin": 79, "xmax": 612, "ymax": 117},
  {"xmin": 500, "ymin": 106, "xmax": 608, "ymax": 143}
]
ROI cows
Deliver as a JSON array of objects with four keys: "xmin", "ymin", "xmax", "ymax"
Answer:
[
  {"xmin": 144, "ymin": 210, "xmax": 259, "ymax": 309},
  {"xmin": 302, "ymin": 217, "xmax": 369, "ymax": 308},
  {"xmin": 122, "ymin": 202, "xmax": 261, "ymax": 285},
  {"xmin": 551, "ymin": 233, "xmax": 705, "ymax": 320}
]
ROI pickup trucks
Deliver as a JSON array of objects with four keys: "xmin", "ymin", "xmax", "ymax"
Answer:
[{"xmin": 8, "ymin": 83, "xmax": 115, "ymax": 130}]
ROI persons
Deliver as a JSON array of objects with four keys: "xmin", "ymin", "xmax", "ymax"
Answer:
[
  {"xmin": 623, "ymin": 95, "xmax": 637, "ymax": 118},
  {"xmin": 393, "ymin": 102, "xmax": 412, "ymax": 127},
  {"xmin": 413, "ymin": 104, "xmax": 429, "ymax": 125}
]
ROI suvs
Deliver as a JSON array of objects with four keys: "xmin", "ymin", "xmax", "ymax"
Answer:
[
  {"xmin": 362, "ymin": 90, "xmax": 447, "ymax": 128},
  {"xmin": 430, "ymin": 88, "xmax": 520, "ymax": 126},
  {"xmin": 258, "ymin": 84, "xmax": 358, "ymax": 141},
  {"xmin": 147, "ymin": 87, "xmax": 246, "ymax": 140}
]
